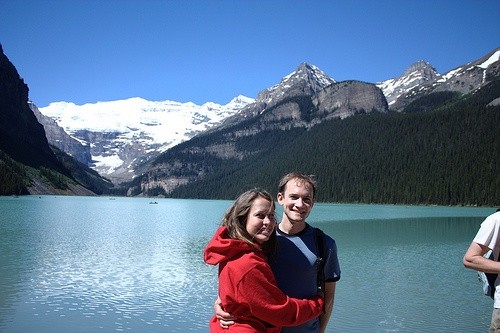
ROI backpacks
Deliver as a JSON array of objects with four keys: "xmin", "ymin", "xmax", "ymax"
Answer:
[{"xmin": 476, "ymin": 207, "xmax": 500, "ymax": 300}]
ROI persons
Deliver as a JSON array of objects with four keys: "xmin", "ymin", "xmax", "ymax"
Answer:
[
  {"xmin": 203, "ymin": 187, "xmax": 325, "ymax": 333},
  {"xmin": 463, "ymin": 209, "xmax": 500, "ymax": 333},
  {"xmin": 212, "ymin": 173, "xmax": 341, "ymax": 332}
]
